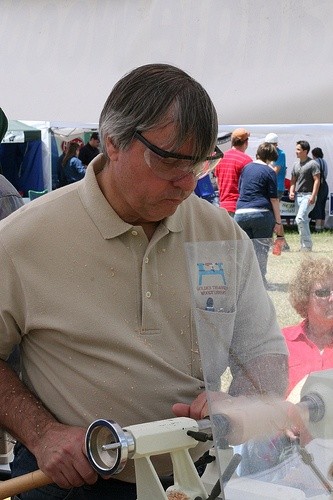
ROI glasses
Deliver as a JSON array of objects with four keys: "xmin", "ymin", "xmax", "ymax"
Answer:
[
  {"xmin": 310, "ymin": 288, "xmax": 333, "ymax": 298},
  {"xmin": 134, "ymin": 132, "xmax": 224, "ymax": 182}
]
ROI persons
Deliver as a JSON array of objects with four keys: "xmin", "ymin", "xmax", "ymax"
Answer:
[
  {"xmin": 0, "ymin": 106, "xmax": 333, "ymax": 476},
  {"xmin": 0, "ymin": 64, "xmax": 290, "ymax": 500}
]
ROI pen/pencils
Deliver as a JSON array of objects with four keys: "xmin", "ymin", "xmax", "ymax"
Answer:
[{"xmin": 205, "ymin": 296, "xmax": 215, "ymax": 311}]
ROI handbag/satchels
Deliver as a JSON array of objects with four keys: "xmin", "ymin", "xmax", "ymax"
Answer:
[{"xmin": 319, "ymin": 180, "xmax": 328, "ymax": 202}]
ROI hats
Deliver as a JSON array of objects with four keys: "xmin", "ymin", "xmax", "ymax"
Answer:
[
  {"xmin": 265, "ymin": 133, "xmax": 278, "ymax": 143},
  {"xmin": 231, "ymin": 128, "xmax": 250, "ymax": 142}
]
